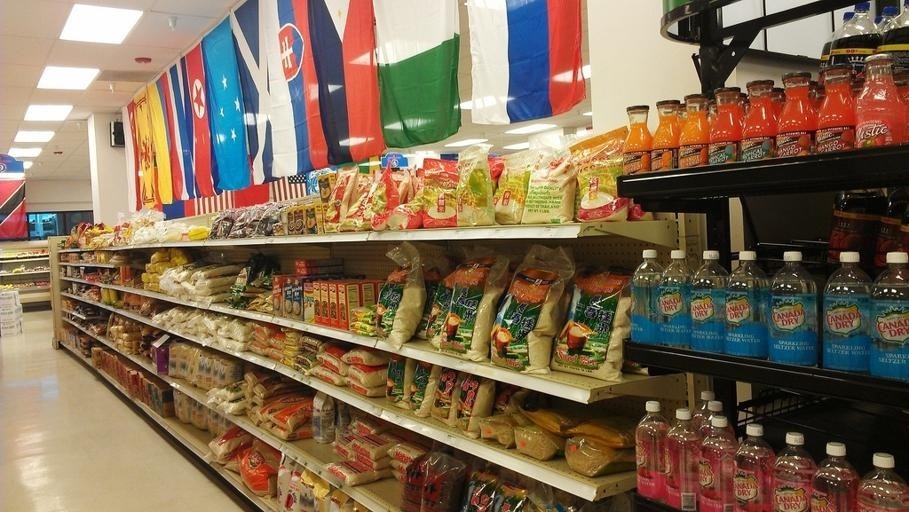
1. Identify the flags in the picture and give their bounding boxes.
[374,0,461,149]
[467,0,587,123]
[292,0,385,171]
[202,14,251,195]
[127,44,224,211]
[165,167,337,221]
[232,0,316,185]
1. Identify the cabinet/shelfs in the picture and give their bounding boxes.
[49,211,707,511]
[616,0,909,512]
[0,240,51,303]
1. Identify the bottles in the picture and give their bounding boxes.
[821,0,908,83]
[628,246,909,381]
[635,390,909,512]
[828,187,909,265]
[618,51,908,178]
